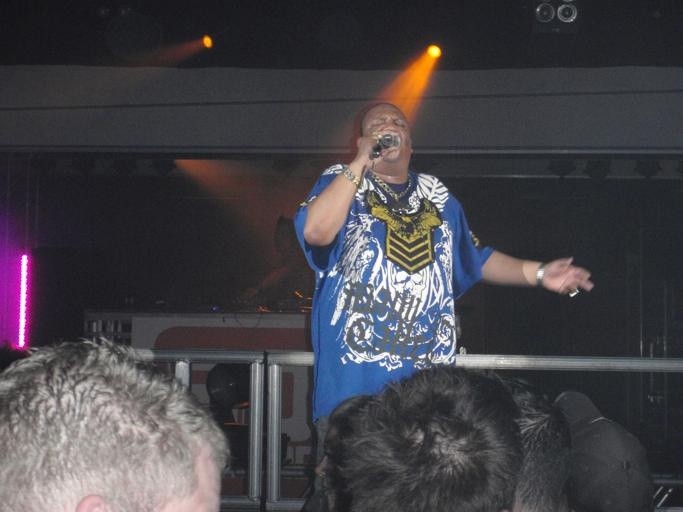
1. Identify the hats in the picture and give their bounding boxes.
[554,391,654,512]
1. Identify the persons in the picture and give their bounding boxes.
[1,337,230,512]
[294,103,596,512]
[302,367,654,512]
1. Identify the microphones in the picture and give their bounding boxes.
[381,133,399,147]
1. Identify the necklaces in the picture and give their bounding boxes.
[372,174,413,201]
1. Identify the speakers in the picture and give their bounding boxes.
[505,0,580,37]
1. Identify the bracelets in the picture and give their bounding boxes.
[341,168,360,189]
[535,262,547,287]
